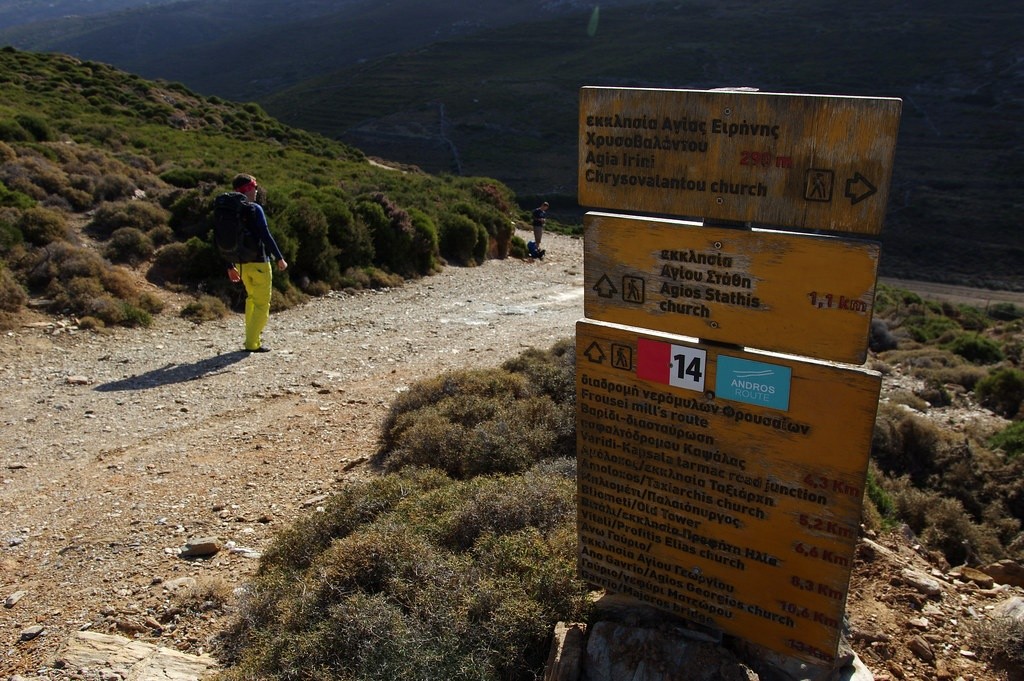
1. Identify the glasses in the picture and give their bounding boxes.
[253,185,258,190]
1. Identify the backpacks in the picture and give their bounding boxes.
[211,190,263,265]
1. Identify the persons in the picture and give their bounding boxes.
[213,173,287,352]
[532,202,549,251]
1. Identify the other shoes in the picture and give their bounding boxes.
[245,346,271,352]
